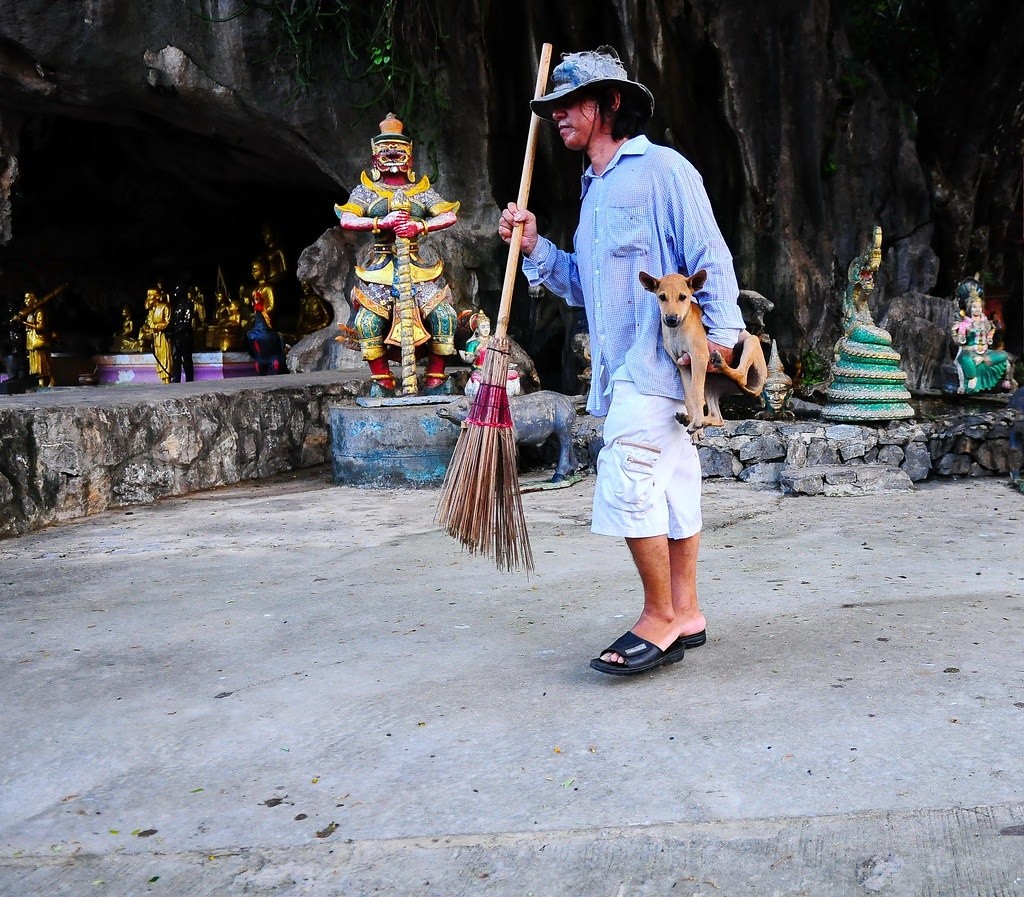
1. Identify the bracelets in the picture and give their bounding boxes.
[418,220,429,236]
[371,217,380,234]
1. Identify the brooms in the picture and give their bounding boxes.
[430,45,555,581]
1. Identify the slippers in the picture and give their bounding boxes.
[590,631,685,676]
[680,630,706,650]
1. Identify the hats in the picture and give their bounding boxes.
[529,45,655,123]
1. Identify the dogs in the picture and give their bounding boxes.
[637,270,768,444]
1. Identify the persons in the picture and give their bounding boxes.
[755,339,794,421]
[332,112,461,399]
[459,309,518,387]
[496,44,745,676]
[952,278,1015,393]
[13,292,56,386]
[115,253,331,385]
